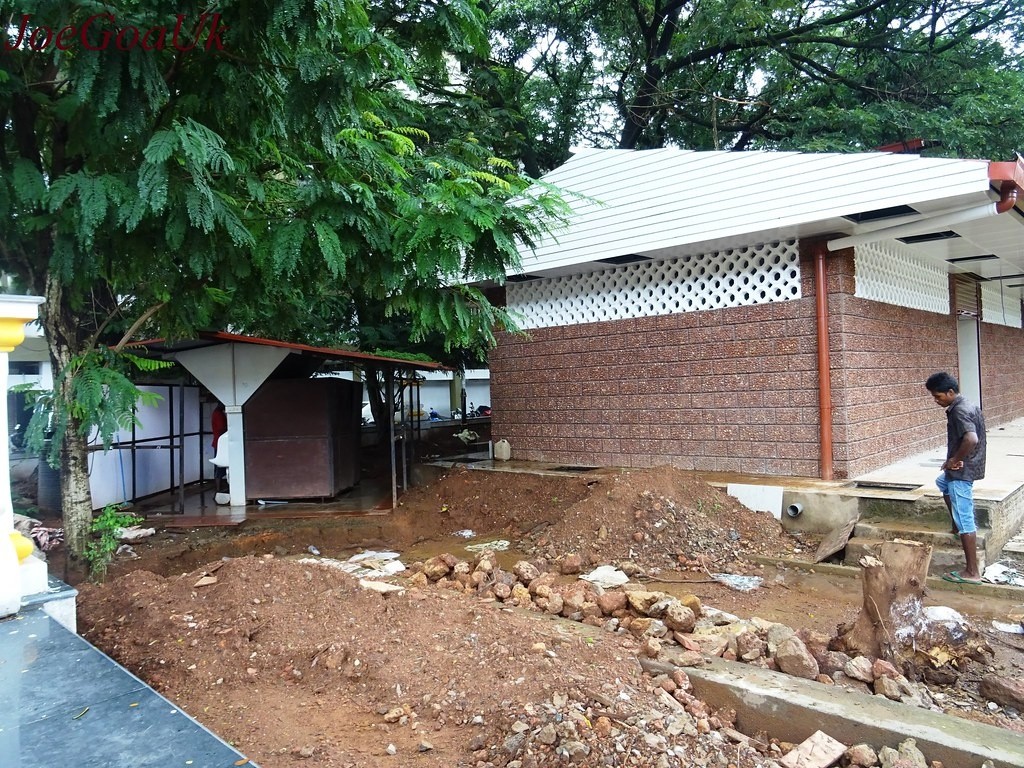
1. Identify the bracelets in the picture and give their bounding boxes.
[949,458,956,467]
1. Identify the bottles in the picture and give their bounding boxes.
[493,438,510,460]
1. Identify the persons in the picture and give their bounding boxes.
[925,372,986,585]
[212,401,227,494]
[9,424,27,454]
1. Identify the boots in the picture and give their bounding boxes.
[216,477,226,493]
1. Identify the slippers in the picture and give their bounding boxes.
[943,572,982,585]
[954,534,959,540]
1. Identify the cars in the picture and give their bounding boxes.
[361,399,492,426]
[9,422,42,488]
